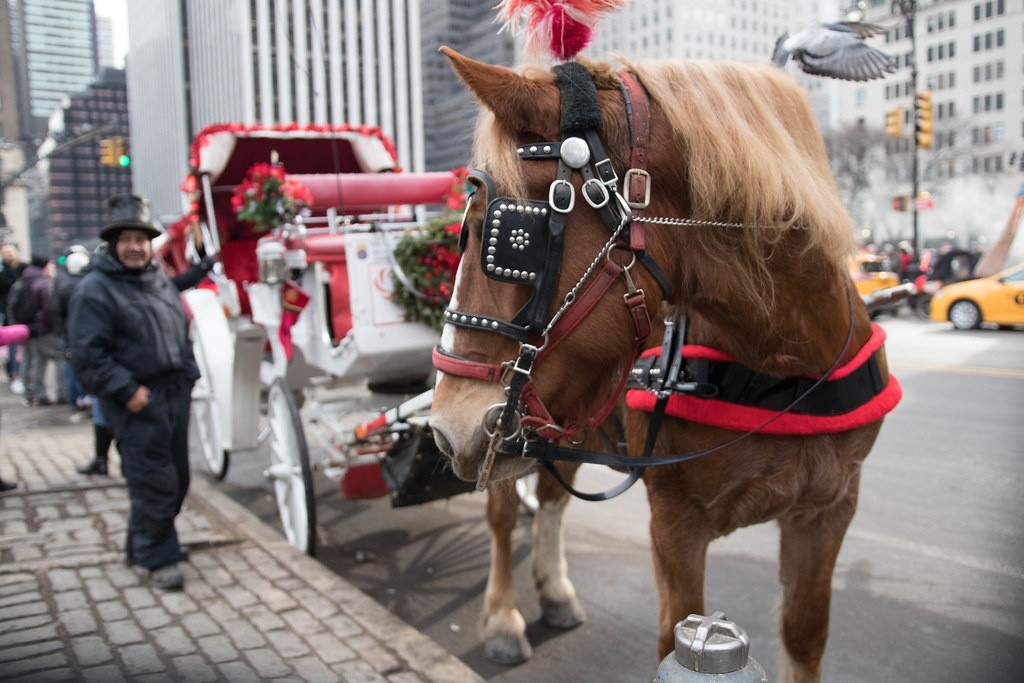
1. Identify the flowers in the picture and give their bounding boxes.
[388,166,481,326]
[231,162,289,234]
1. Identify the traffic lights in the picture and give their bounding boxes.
[888,91,933,147]
[100,138,131,168]
[57,248,66,272]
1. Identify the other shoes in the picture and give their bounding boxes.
[166,544,189,566]
[77,457,108,476]
[130,558,183,590]
[0,478,18,493]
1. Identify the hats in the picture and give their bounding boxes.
[96,194,158,242]
[33,254,48,266]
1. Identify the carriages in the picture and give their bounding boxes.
[151,41,891,683]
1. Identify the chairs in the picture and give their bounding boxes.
[199,187,293,355]
[294,170,485,344]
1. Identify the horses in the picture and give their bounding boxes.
[425,45,891,683]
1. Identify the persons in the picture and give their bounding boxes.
[842,231,985,316]
[0,237,227,491]
[65,192,201,594]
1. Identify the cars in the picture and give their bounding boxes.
[927,262,1024,330]
[844,254,899,316]
